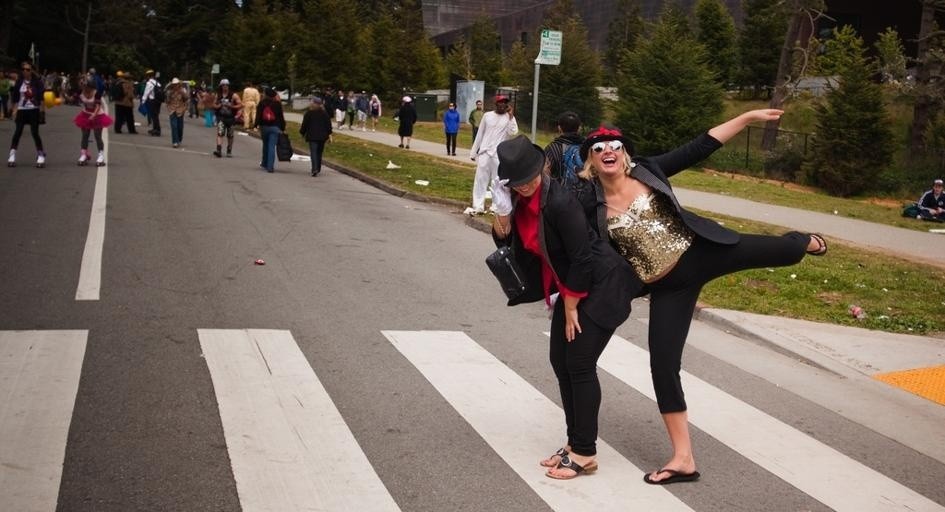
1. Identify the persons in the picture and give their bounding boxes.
[489,134,648,479]
[1,60,385,178]
[470,93,522,217]
[573,106,829,487]
[916,178,944,224]
[444,101,459,157]
[469,100,485,147]
[394,95,417,149]
[544,110,587,196]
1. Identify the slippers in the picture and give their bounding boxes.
[541,447,598,480]
[645,469,699,483]
[807,234,826,256]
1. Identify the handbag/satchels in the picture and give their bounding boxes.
[904,204,921,217]
[277,134,293,161]
[486,235,527,301]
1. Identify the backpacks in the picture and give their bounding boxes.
[150,80,164,103]
[562,144,598,209]
[109,81,127,101]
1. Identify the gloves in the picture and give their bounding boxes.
[489,176,513,217]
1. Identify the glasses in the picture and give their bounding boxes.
[592,140,623,153]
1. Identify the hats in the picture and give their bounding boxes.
[403,97,411,102]
[220,79,229,85]
[580,127,635,163]
[264,88,277,97]
[172,78,180,85]
[933,180,943,185]
[497,135,546,187]
[495,95,509,103]
[310,98,321,103]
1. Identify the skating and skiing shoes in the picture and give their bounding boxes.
[77,150,87,165]
[96,151,105,167]
[8,149,16,167]
[36,152,45,167]
